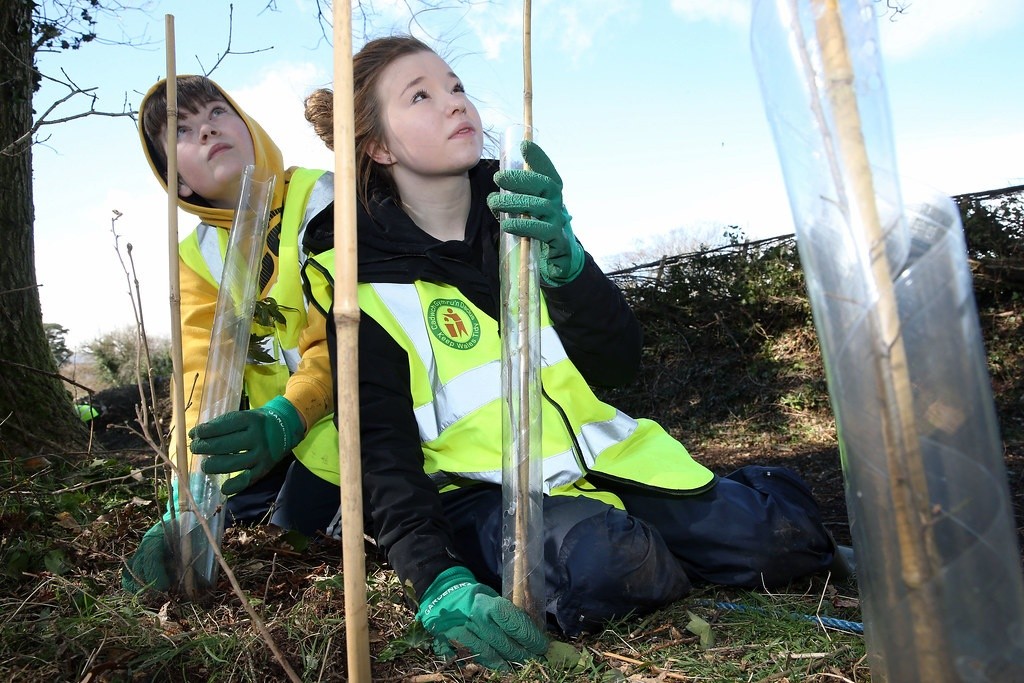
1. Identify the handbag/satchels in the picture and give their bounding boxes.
[723,466,836,595]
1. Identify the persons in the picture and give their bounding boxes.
[308,36,850,664]
[125,77,343,605]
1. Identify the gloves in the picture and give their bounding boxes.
[188,394,306,496]
[121,474,217,599]
[483,140,586,288]
[413,565,549,673]
